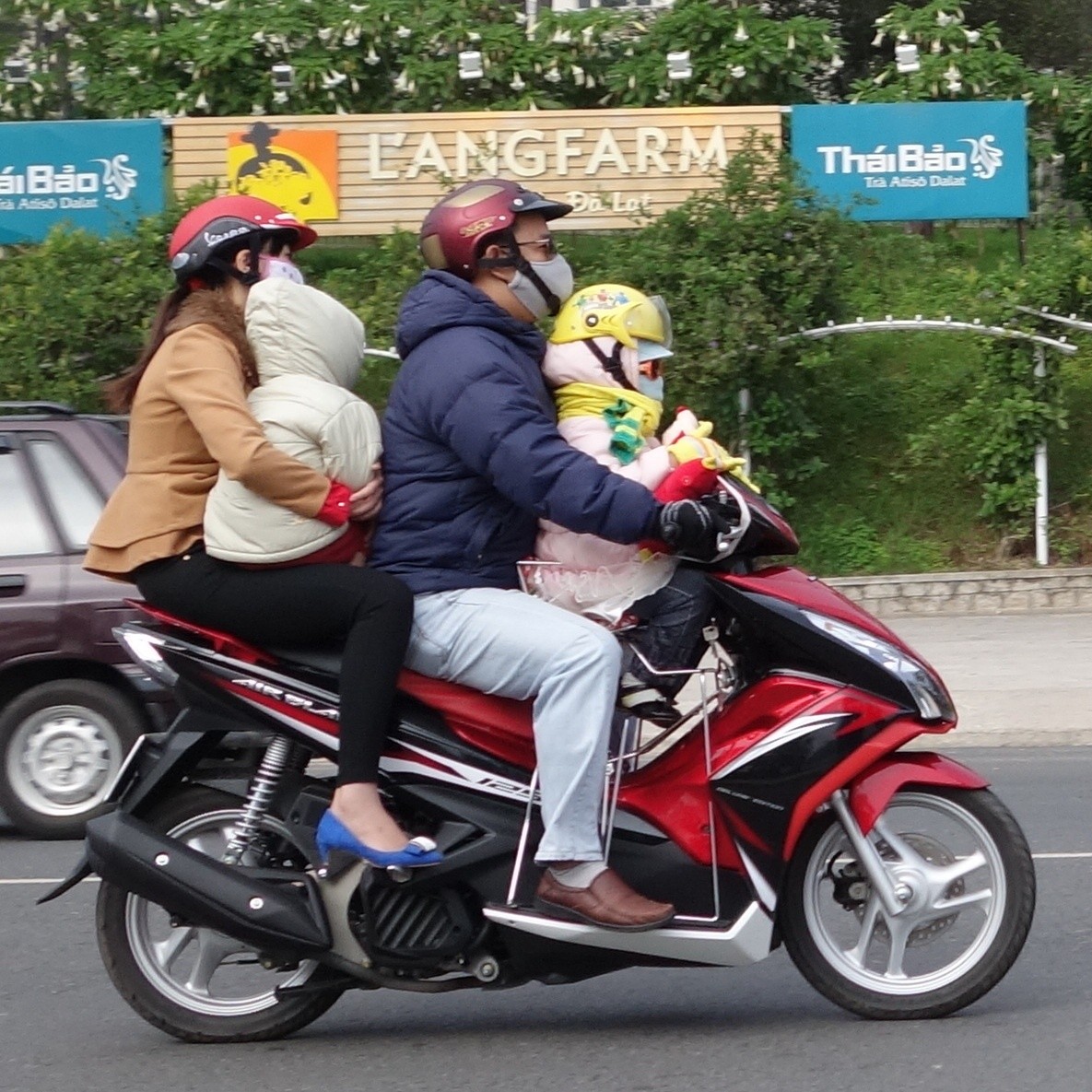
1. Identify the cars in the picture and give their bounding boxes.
[0,399,172,846]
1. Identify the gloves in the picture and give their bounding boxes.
[654,498,731,554]
[667,416,747,474]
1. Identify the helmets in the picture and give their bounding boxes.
[420,178,574,282]
[169,195,319,284]
[549,283,666,350]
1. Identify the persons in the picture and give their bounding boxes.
[527,284,730,729]
[203,276,385,565]
[83,199,443,868]
[369,179,731,932]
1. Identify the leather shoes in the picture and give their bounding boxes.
[616,677,682,731]
[533,868,678,934]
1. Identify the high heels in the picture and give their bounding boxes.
[315,808,447,869]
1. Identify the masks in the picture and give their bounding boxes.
[229,255,306,288]
[638,375,668,403]
[490,248,576,320]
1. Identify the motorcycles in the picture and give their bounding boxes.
[35,464,1040,1049]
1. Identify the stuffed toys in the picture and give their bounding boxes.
[663,407,760,493]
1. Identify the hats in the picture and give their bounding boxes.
[635,338,674,363]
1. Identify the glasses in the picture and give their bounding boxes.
[476,238,556,261]
